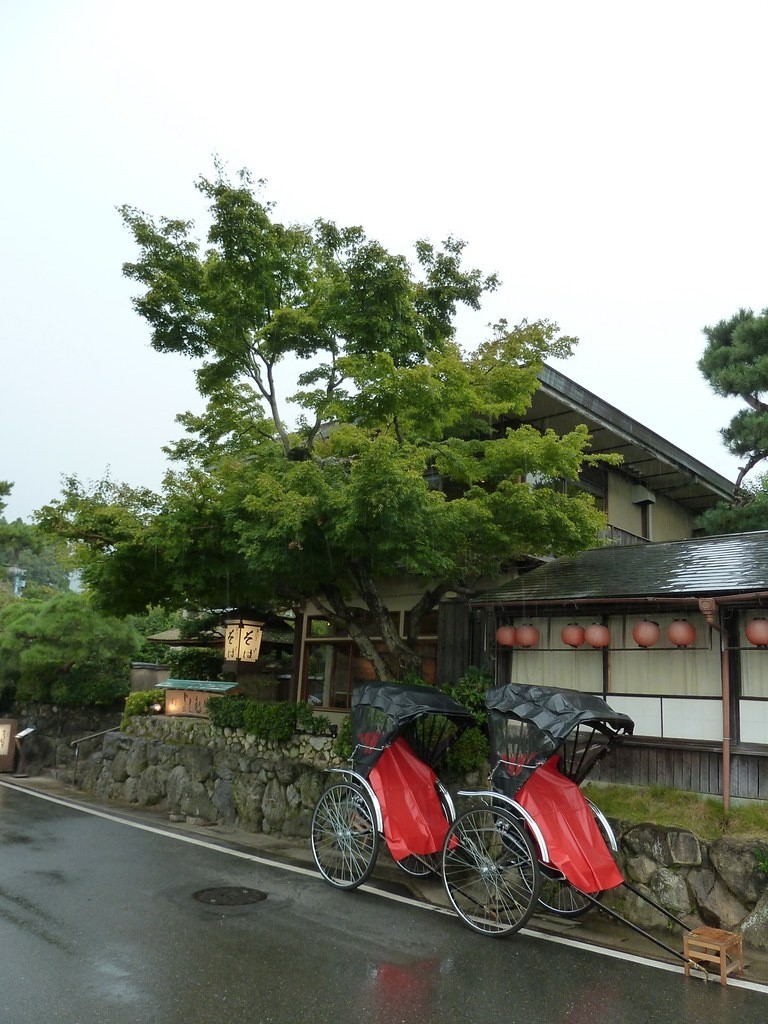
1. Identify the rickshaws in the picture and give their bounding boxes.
[438,682,745,981]
[308,677,625,929]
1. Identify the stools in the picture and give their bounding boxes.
[683,925,745,986]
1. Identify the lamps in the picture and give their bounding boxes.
[220,603,267,664]
[497,614,516,649]
[585,613,612,647]
[516,614,539,648]
[632,613,660,648]
[668,613,696,648]
[746,607,768,649]
[149,703,162,712]
[562,615,585,649]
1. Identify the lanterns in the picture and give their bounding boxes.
[745,617,768,647]
[667,618,696,647]
[515,622,540,647]
[561,623,586,648]
[585,622,610,648]
[632,619,660,648]
[496,623,517,647]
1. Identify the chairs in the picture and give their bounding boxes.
[500,752,584,852]
[356,730,434,823]
